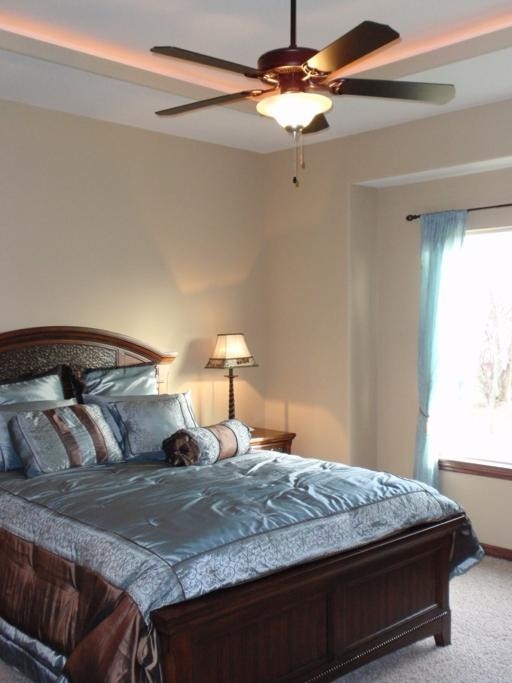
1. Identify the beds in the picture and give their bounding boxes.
[0,325,484,683]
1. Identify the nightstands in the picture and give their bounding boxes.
[243,427,296,454]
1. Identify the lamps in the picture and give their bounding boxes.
[205,333,258,419]
[255,92,332,184]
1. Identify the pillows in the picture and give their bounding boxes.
[0,363,252,477]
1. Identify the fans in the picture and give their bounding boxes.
[150,0,455,133]
